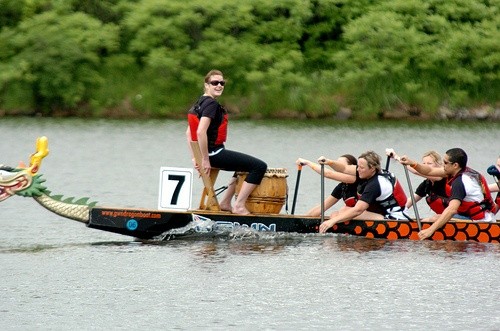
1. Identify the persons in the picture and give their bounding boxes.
[296,147,500,242]
[186,69,268,214]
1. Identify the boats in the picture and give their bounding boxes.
[0,135,500,244]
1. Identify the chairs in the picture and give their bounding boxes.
[190,140,236,213]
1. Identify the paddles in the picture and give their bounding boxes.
[386,153,393,170]
[291,162,304,215]
[321,159,325,224]
[492,168,500,189]
[402,158,422,231]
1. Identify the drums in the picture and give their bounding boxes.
[237,168,289,215]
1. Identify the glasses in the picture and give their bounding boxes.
[443,160,455,164]
[206,80,225,86]
